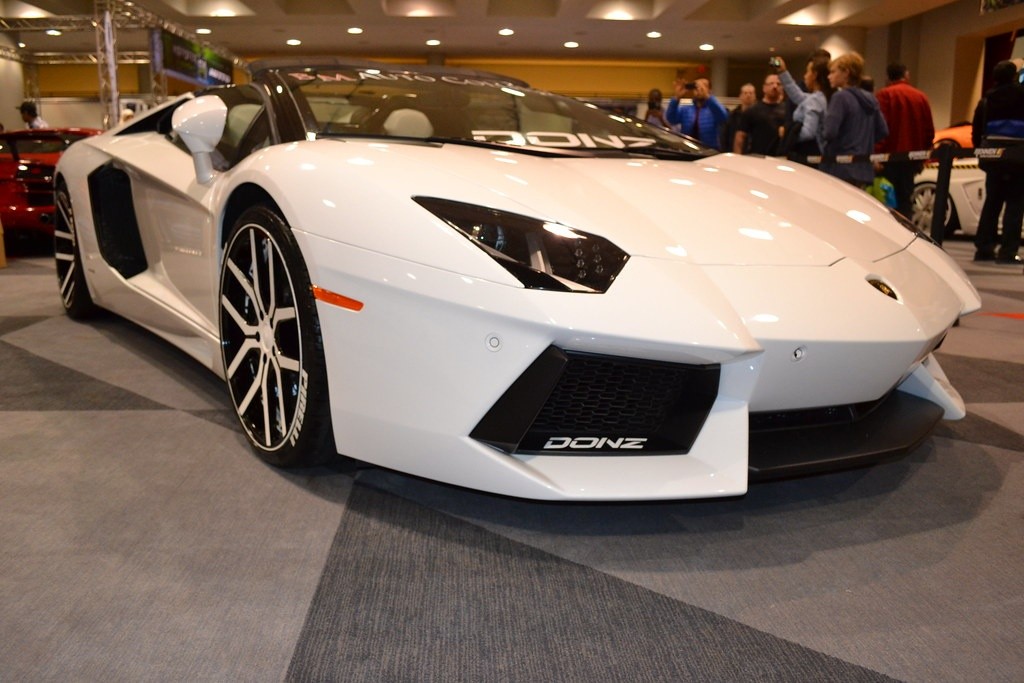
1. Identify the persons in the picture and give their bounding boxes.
[645,90,676,131]
[719,48,890,193]
[874,62,934,221]
[971,59,1024,265]
[666,78,729,151]
[15,101,49,131]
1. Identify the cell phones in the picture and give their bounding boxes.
[685,83,696,90]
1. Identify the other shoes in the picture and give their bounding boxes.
[973,249,1023,264]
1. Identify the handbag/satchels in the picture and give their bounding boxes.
[862,167,898,211]
[978,134,1024,174]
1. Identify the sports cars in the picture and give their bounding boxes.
[52,62,983,502]
[0,128,108,253]
[910,121,1024,239]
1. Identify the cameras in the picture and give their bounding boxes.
[770,57,780,67]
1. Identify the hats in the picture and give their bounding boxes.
[15,101,36,110]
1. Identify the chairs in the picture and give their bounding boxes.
[228,104,269,151]
[383,108,435,139]
